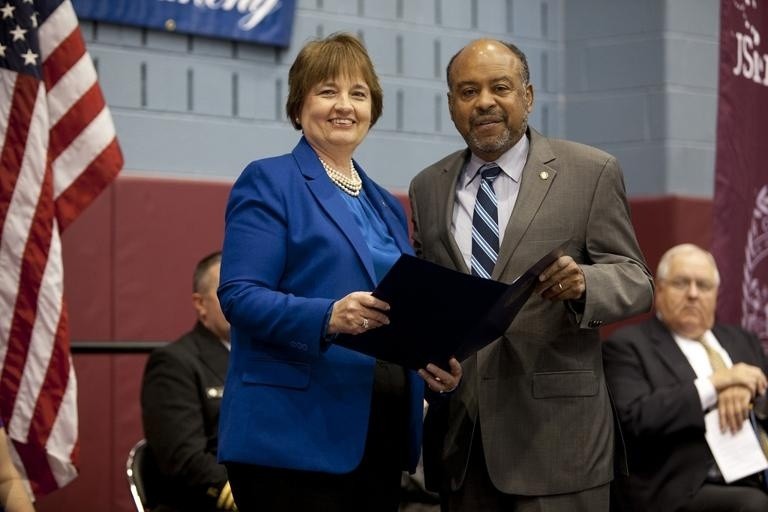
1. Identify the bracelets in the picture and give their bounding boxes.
[0,476,22,483]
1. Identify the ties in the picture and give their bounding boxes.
[469,167,504,279]
[696,335,767,460]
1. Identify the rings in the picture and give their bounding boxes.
[362,317,370,328]
[557,282,564,292]
[439,387,445,394]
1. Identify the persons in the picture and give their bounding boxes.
[0,425,37,511]
[138,250,245,510]
[406,34,656,511]
[600,235,768,511]
[222,27,467,511]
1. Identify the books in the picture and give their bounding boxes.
[703,402,768,486]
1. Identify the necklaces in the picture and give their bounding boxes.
[317,154,363,198]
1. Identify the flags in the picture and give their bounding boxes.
[0,2,125,494]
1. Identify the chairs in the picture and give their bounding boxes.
[126,437,150,512]
